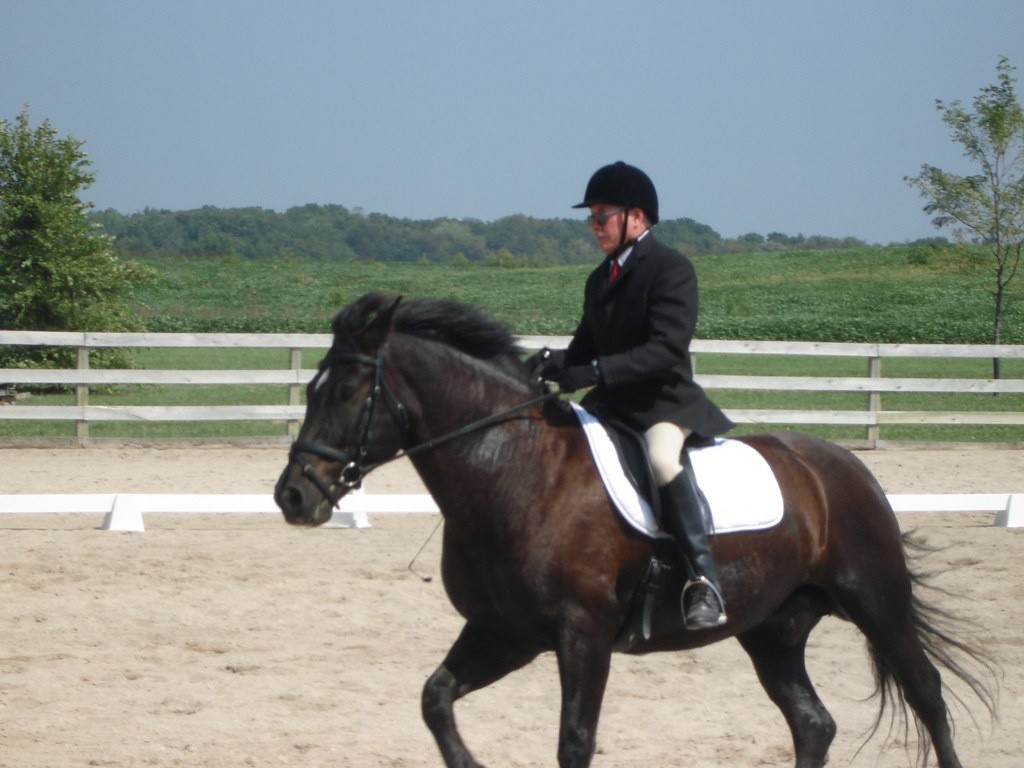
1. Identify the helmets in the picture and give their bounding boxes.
[571,161,658,213]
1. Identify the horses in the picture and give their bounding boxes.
[272,289,1007,768]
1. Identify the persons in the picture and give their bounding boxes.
[525,162,737,629]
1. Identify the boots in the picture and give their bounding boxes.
[656,468,723,630]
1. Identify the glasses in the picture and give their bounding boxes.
[587,207,625,226]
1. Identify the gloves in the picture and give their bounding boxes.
[525,348,600,393]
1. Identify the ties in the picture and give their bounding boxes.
[608,261,620,286]
